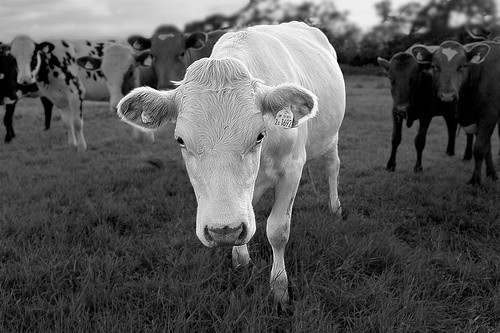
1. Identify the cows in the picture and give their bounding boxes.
[377,42,470,172]
[5,34,130,152]
[412,39,500,188]
[120,50,165,144]
[116,20,347,312]
[128,25,242,91]
[0,39,55,140]
